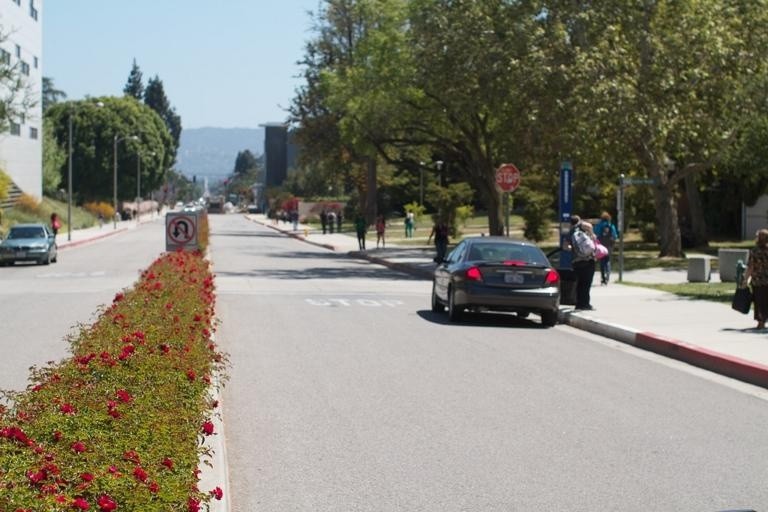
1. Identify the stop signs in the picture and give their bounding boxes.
[495,161,521,192]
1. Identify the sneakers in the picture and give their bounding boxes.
[575,304,596,312]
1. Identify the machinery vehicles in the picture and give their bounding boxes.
[206,196,224,214]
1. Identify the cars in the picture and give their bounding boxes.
[184,204,195,212]
[0,224,57,266]
[432,236,579,326]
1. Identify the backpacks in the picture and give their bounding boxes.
[571,231,595,258]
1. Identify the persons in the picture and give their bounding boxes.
[97,212,104,230]
[561,213,598,311]
[374,210,387,251]
[50,210,60,237]
[427,215,450,266]
[273,206,344,235]
[353,211,369,251]
[404,209,417,238]
[739,229,768,330]
[592,211,618,285]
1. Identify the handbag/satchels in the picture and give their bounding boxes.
[594,238,609,261]
[731,286,753,315]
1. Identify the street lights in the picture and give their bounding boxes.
[435,159,444,214]
[68,102,104,241]
[114,136,138,229]
[137,152,156,222]
[419,160,426,206]
